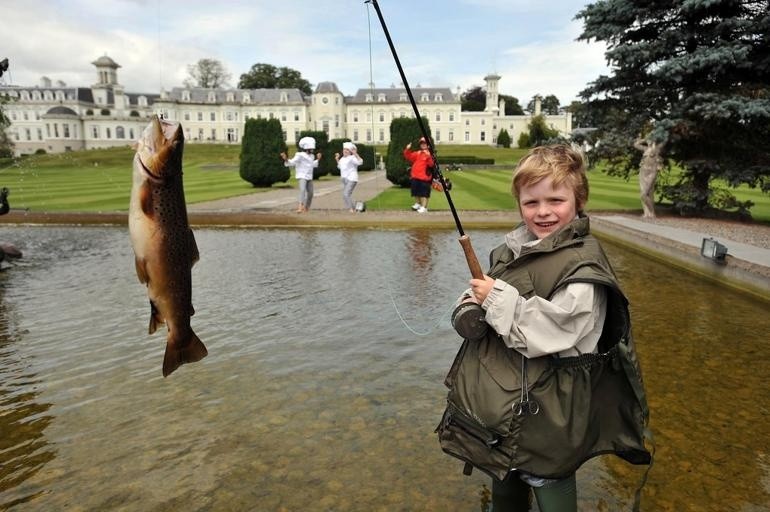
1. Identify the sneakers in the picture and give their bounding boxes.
[411,202,422,210]
[303,207,309,214]
[416,207,427,214]
[350,207,357,216]
[296,202,303,213]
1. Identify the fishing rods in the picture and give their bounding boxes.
[365,0,495,338]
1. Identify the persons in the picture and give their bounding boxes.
[280,136,322,212]
[451,144,653,511]
[336,141,363,212]
[404,136,435,213]
[632,131,669,218]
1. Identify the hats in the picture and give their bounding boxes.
[419,137,426,143]
[342,141,357,154]
[299,137,316,150]
